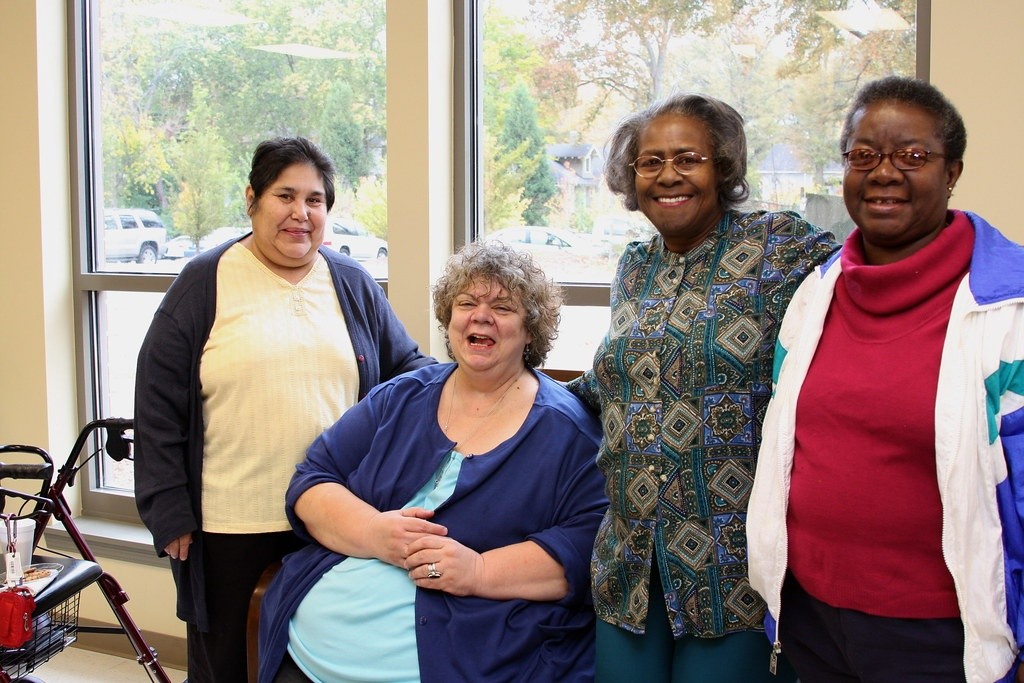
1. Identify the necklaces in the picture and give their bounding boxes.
[434,366,512,489]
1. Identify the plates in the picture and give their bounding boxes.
[0,563,64,586]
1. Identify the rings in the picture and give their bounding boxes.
[427,563,441,578]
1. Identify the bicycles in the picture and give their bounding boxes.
[1,415,174,683]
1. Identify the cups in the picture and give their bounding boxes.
[0,519,36,570]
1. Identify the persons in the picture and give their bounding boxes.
[557,90,842,683]
[134,135,442,683]
[256,239,609,683]
[743,73,1024,683]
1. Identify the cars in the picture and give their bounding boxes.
[161,235,195,262]
[198,226,254,254]
[482,228,611,282]
[321,218,388,260]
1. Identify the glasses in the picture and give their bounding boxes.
[629,152,716,178]
[842,148,949,171]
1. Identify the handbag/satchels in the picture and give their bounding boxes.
[0,584,36,649]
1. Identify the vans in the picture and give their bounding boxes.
[105,208,167,266]
